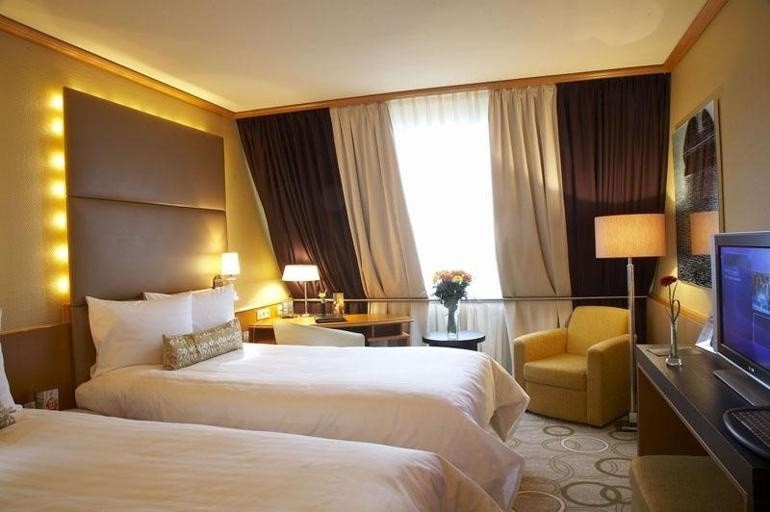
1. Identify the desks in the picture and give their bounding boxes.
[247,313,415,347]
[635,344,769,512]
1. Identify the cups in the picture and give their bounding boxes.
[333,292,345,316]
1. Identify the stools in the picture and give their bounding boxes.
[629,455,744,511]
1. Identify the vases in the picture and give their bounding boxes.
[445,305,458,339]
[665,323,682,366]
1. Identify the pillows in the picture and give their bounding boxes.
[85,291,193,378]
[161,318,243,370]
[143,283,235,336]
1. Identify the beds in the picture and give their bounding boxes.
[1,408,503,512]
[74,342,531,511]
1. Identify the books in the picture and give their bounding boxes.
[315,315,346,322]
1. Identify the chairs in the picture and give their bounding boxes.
[272,319,365,347]
[512,305,638,428]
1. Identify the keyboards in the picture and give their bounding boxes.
[722,407,770,459]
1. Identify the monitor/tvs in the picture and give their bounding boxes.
[710,231,770,408]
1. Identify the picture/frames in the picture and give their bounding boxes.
[670,93,724,291]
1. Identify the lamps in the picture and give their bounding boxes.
[594,213,668,433]
[222,252,240,281]
[282,264,320,318]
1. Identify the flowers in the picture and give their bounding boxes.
[660,275,680,358]
[430,269,472,333]
